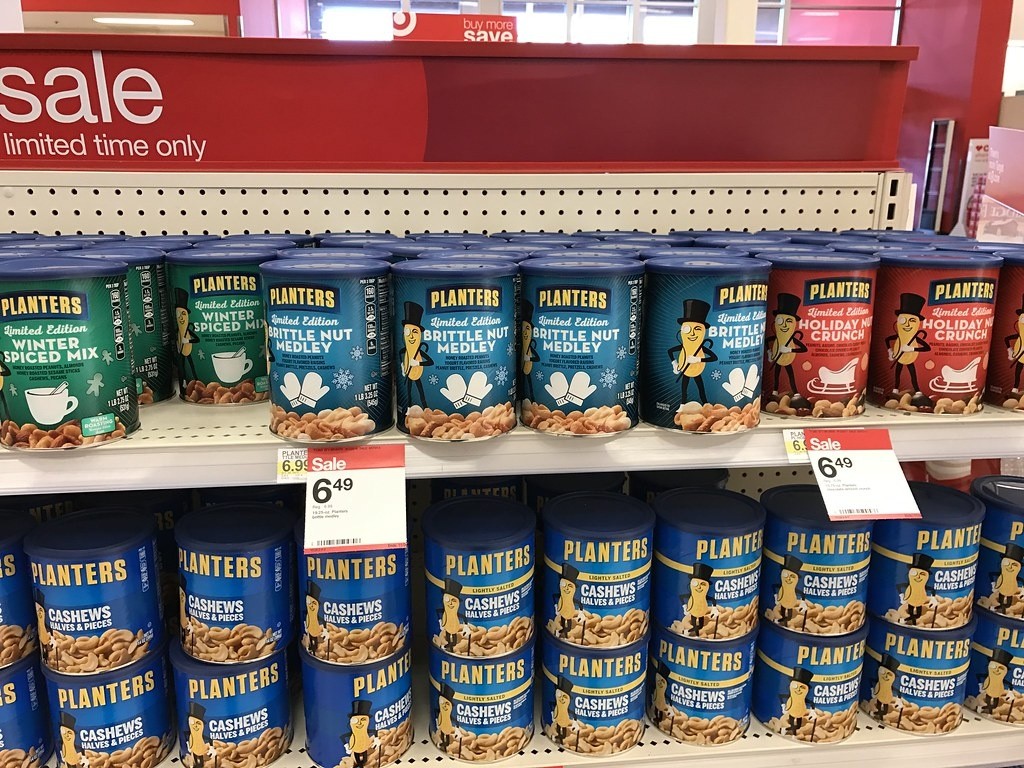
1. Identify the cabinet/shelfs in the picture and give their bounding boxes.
[0,164,1024,768]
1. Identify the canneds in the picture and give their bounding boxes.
[0,231,1024,768]
